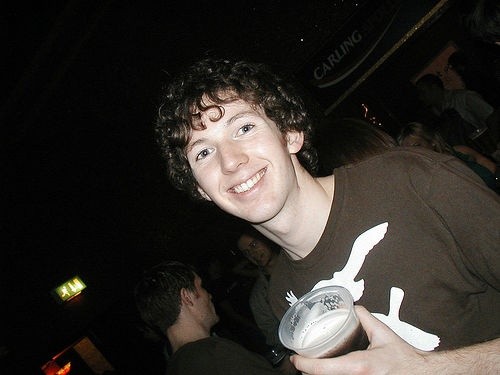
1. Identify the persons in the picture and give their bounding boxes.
[399,121,499,195]
[412,74,495,154]
[235,232,282,347]
[126,259,277,375]
[155,58,500,375]
[314,115,397,172]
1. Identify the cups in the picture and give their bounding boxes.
[279,285,371,359]
